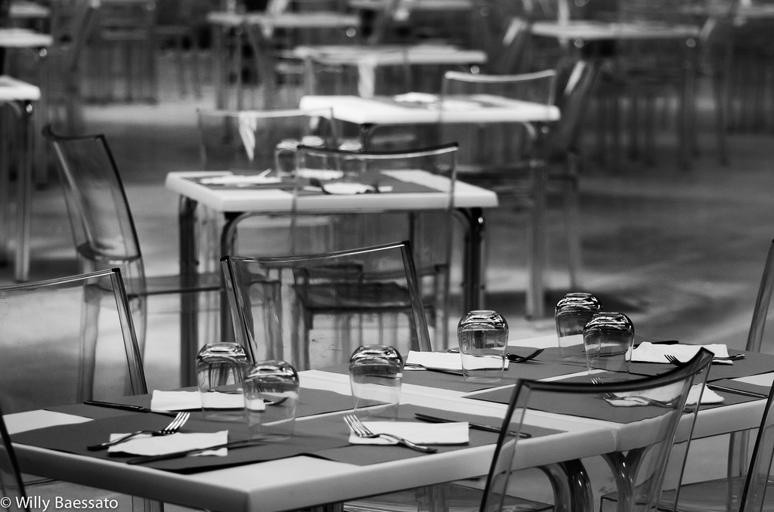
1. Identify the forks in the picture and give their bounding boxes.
[447,347,546,363]
[663,351,746,367]
[341,414,439,457]
[587,375,686,410]
[84,410,193,453]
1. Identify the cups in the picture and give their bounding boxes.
[348,342,402,423]
[456,307,507,385]
[273,145,367,187]
[586,310,637,385]
[196,340,250,424]
[553,289,603,364]
[240,359,301,446]
